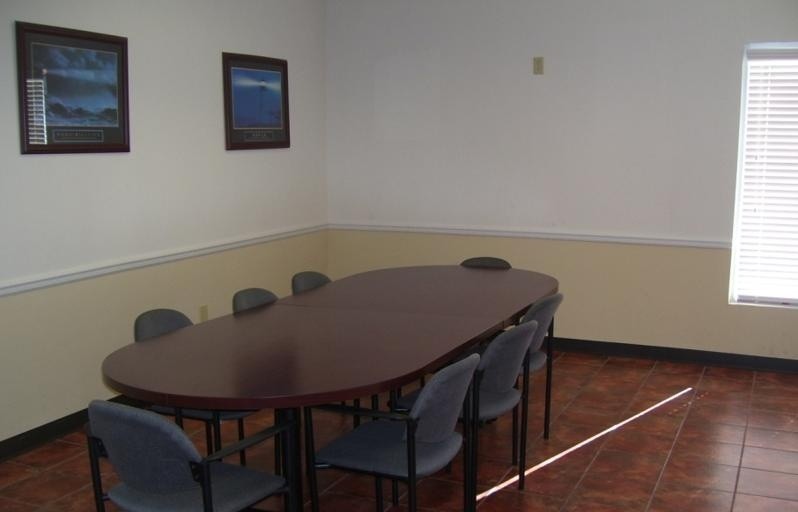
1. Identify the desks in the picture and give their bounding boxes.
[102,265,558,511]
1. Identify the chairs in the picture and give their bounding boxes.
[86,398,299,511]
[386,321,539,492]
[493,292,565,466]
[133,307,249,470]
[232,288,320,482]
[314,353,482,511]
[460,256,513,268]
[292,270,404,423]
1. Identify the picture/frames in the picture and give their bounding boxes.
[223,49,292,150]
[16,20,132,155]
